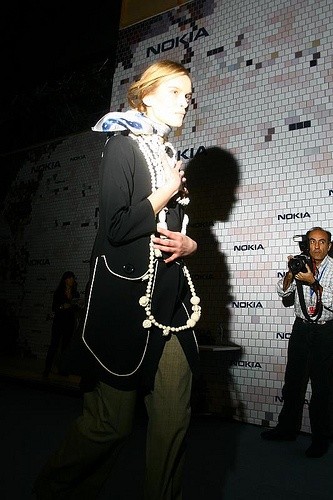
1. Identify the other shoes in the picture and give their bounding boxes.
[305,444,328,457]
[260,429,294,442]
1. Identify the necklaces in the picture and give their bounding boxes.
[127,133,201,335]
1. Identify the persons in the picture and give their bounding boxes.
[42,270,84,377]
[260,227,333,458]
[31,59,202,500]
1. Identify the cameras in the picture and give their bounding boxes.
[288,235,313,274]
